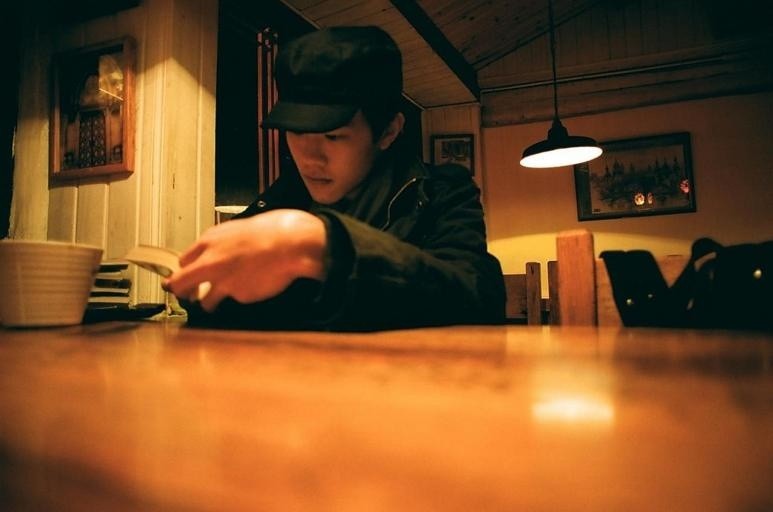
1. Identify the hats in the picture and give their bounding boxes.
[259,25,403,135]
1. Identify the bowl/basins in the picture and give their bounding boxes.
[0,241,103,329]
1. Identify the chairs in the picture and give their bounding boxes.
[505,227,710,326]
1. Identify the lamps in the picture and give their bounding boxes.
[517,1,604,170]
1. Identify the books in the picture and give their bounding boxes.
[125,242,213,301]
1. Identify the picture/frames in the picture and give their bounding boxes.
[49,34,136,181]
[428,131,475,179]
[572,131,697,223]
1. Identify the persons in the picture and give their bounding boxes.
[161,25,506,332]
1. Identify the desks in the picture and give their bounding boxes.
[0,319,772,512]
